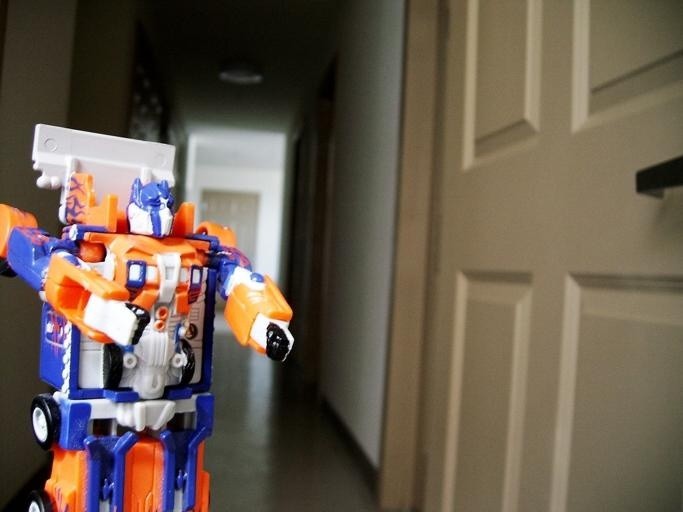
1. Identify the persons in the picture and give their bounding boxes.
[1,122,296,512]
